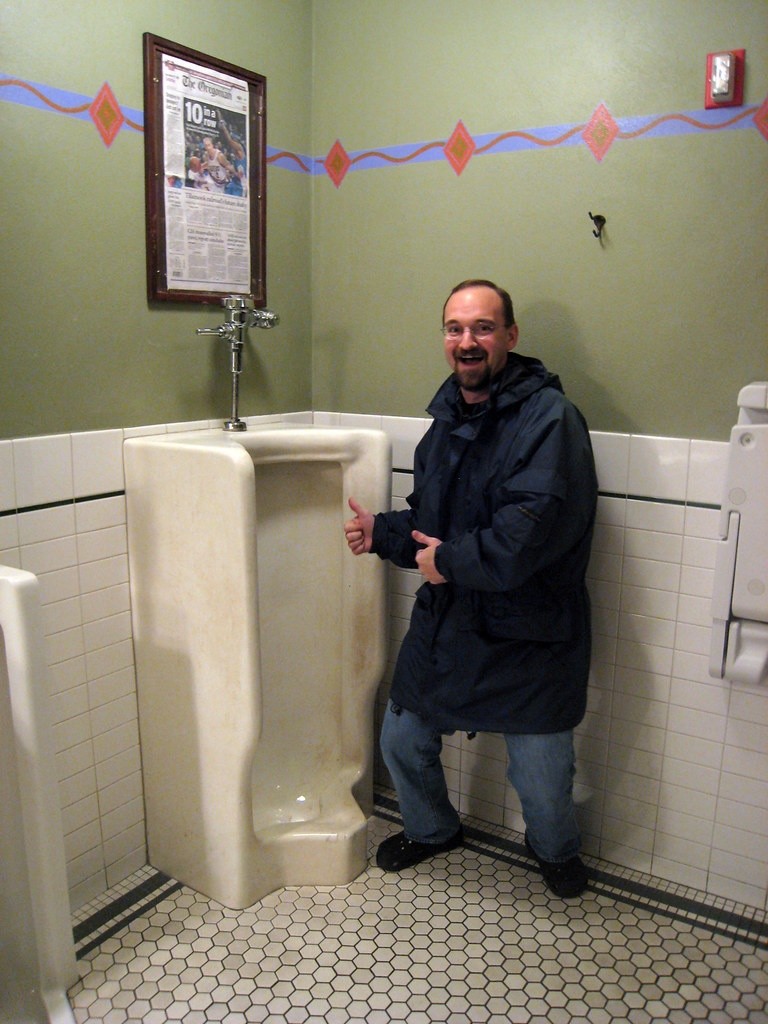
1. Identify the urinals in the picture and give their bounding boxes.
[1,566,81,1023]
[124,426,391,912]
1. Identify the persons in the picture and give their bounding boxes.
[189,110,246,196]
[344,279,598,897]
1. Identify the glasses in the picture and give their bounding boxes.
[440,323,505,340]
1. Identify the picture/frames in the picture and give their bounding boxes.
[141,31,269,311]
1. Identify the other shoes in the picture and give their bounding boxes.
[525,829,588,897]
[376,823,463,872]
